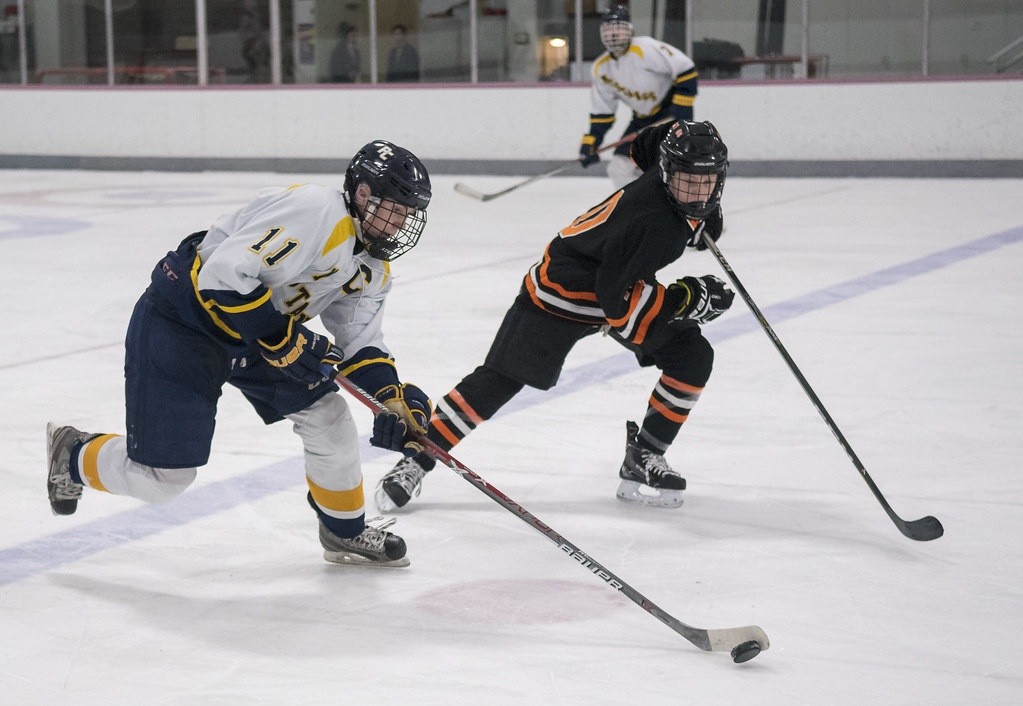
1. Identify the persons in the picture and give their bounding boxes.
[240,0,291,83]
[46,139,432,567]
[331,23,361,83]
[376,119,736,514]
[387,24,420,82]
[579,5,700,188]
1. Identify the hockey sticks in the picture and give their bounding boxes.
[454,115,675,203]
[701,228,945,542]
[319,362,774,656]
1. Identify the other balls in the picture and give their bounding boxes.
[730,640,761,664]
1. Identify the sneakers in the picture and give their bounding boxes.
[616,421,686,509]
[307,492,411,567]
[46,420,106,515]
[374,453,436,514]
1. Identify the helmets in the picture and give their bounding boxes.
[342,140,432,260]
[657,119,730,221]
[602,5,628,22]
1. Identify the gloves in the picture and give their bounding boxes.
[369,383,433,456]
[667,275,735,325]
[579,134,603,167]
[256,314,344,391]
[686,204,723,251]
